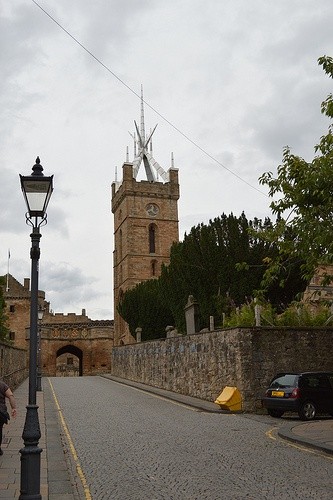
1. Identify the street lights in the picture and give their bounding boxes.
[18,156,54,500]
[36,304,46,391]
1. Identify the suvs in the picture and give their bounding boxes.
[261,370,333,422]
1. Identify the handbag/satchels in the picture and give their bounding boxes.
[3,412,10,424]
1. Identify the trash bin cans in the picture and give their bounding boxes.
[214,385,243,410]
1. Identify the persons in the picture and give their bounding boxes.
[0,381,17,455]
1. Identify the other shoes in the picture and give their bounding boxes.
[0,451,3,455]
